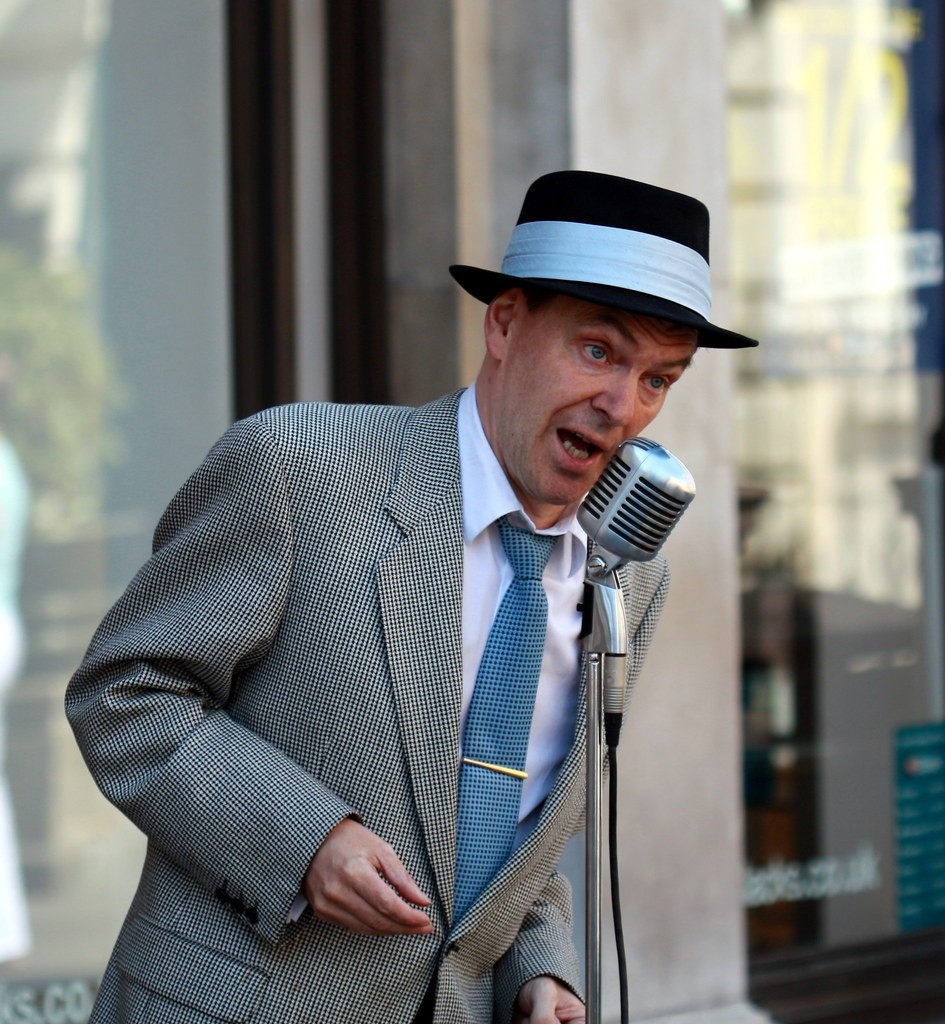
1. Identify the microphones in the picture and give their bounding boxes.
[577,436,697,747]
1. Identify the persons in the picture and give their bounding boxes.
[64,169,758,1024]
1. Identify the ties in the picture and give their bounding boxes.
[453,514,559,930]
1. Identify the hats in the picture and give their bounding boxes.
[449,170,759,349]
